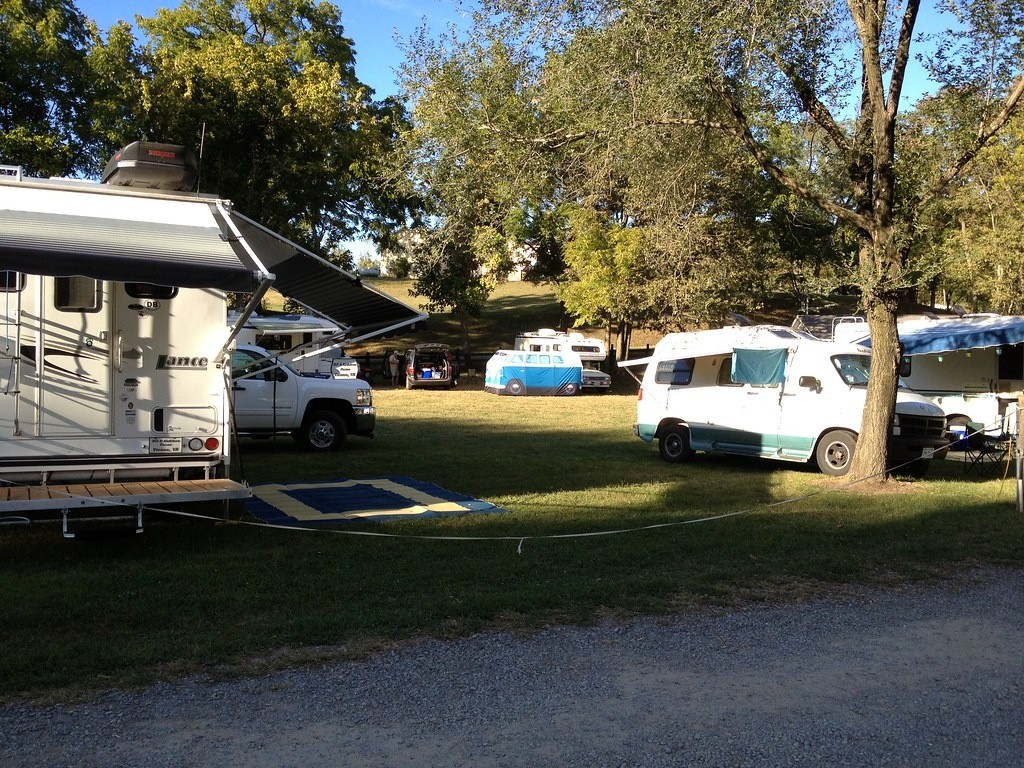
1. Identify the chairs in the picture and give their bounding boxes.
[962,422,1015,477]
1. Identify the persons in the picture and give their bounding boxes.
[389,350,401,389]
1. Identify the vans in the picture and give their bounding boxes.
[617,313,1024,476]
[514,328,611,392]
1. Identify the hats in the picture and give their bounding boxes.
[394,350,400,355]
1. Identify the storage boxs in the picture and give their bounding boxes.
[416,367,442,379]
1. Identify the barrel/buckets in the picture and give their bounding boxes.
[422,368,432,378]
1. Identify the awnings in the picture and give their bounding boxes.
[232,207,429,377]
[1,184,276,366]
[851,317,1024,355]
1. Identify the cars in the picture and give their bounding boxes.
[227,345,377,453]
[383,342,452,390]
